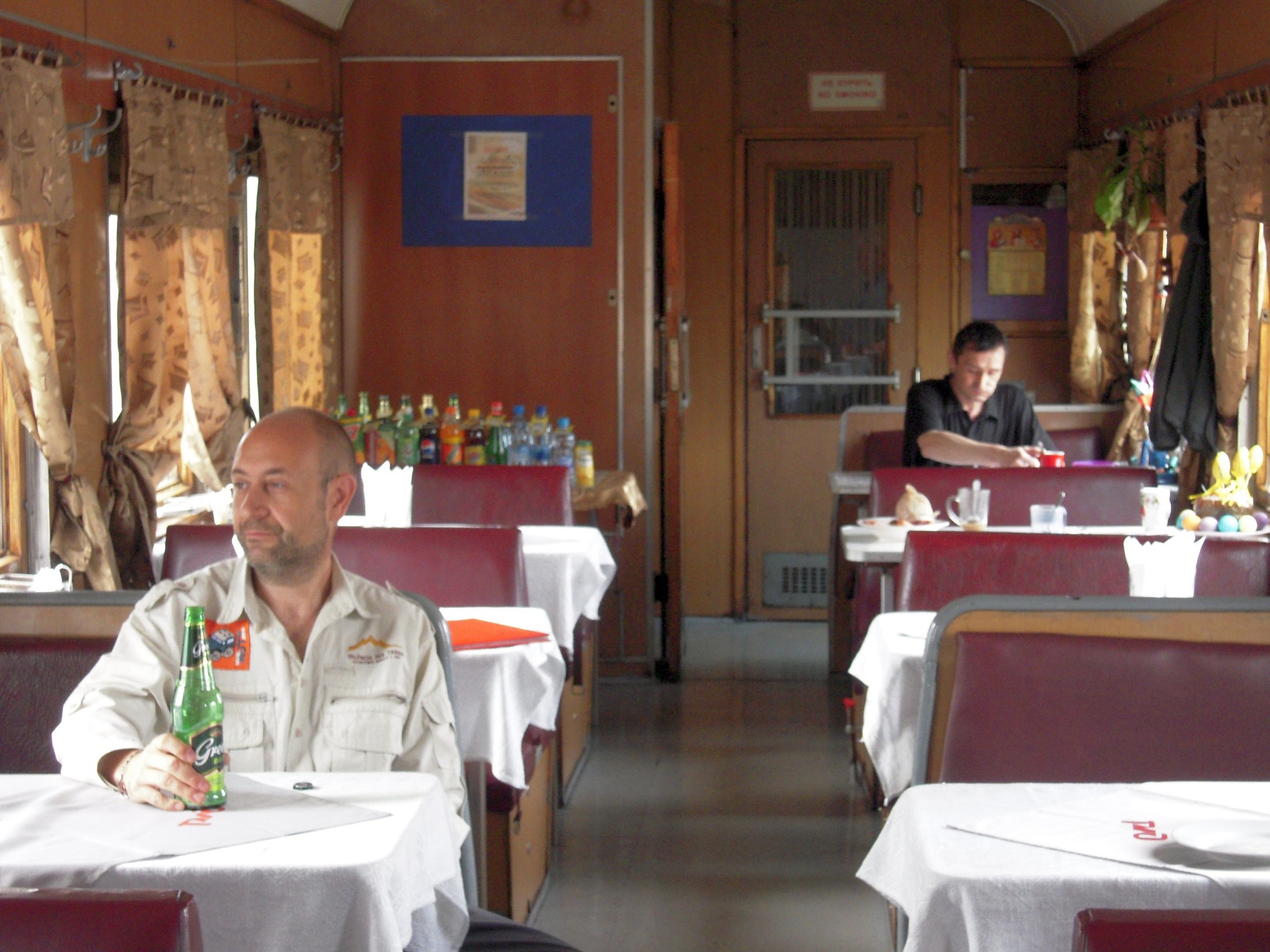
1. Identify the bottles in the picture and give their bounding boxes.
[551,418,577,485]
[485,401,509,465]
[529,405,552,466]
[575,439,595,488]
[171,607,227,809]
[438,393,463,466]
[395,394,417,464]
[418,394,438,463]
[357,390,373,465]
[463,407,485,466]
[509,405,529,467]
[373,393,395,468]
[334,393,357,456]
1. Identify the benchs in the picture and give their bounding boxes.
[0,402,1270,952]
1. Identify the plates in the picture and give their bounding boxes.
[1172,822,1270,865]
[1073,460,1119,466]
[855,516,950,533]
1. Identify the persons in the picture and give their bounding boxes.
[50,405,584,952]
[901,320,1056,467]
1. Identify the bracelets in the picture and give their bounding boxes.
[118,749,144,797]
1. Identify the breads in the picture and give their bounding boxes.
[894,483,934,524]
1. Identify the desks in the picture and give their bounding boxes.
[439,605,566,908]
[850,610,939,810]
[0,769,472,952]
[569,471,646,535]
[854,781,1270,952]
[339,514,614,657]
[840,523,1179,612]
[828,469,876,523]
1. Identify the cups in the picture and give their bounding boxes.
[945,486,991,532]
[1038,451,1066,467]
[1029,503,1069,533]
[1140,487,1172,526]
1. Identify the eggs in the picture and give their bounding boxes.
[1177,509,1268,533]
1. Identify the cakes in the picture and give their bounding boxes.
[1191,446,1263,518]
